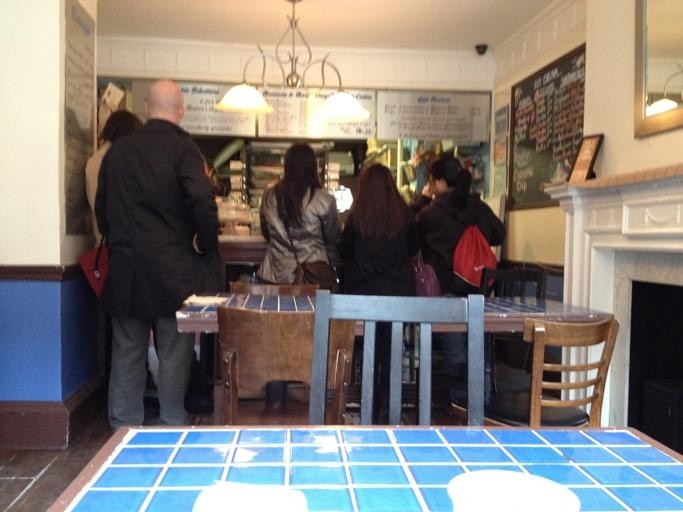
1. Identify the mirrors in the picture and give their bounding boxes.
[631,0,683,139]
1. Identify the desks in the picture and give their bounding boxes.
[199,233,419,386]
[48,425,683,512]
[176,295,364,403]
[432,296,613,397]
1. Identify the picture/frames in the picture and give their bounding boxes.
[566,133,605,183]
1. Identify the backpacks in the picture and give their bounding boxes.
[431,201,497,298]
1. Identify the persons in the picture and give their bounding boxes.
[85,109,144,248]
[408,153,499,414]
[337,164,421,423]
[256,143,342,293]
[94,79,215,428]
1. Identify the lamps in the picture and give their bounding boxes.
[210,0,372,129]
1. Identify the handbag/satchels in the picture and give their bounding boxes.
[78,230,108,295]
[283,219,334,293]
[413,248,441,296]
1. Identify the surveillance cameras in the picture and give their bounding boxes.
[475,44,488,54]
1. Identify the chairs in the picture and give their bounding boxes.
[310,290,485,430]
[231,282,320,296]
[216,311,357,426]
[450,316,620,429]
[456,266,547,394]
